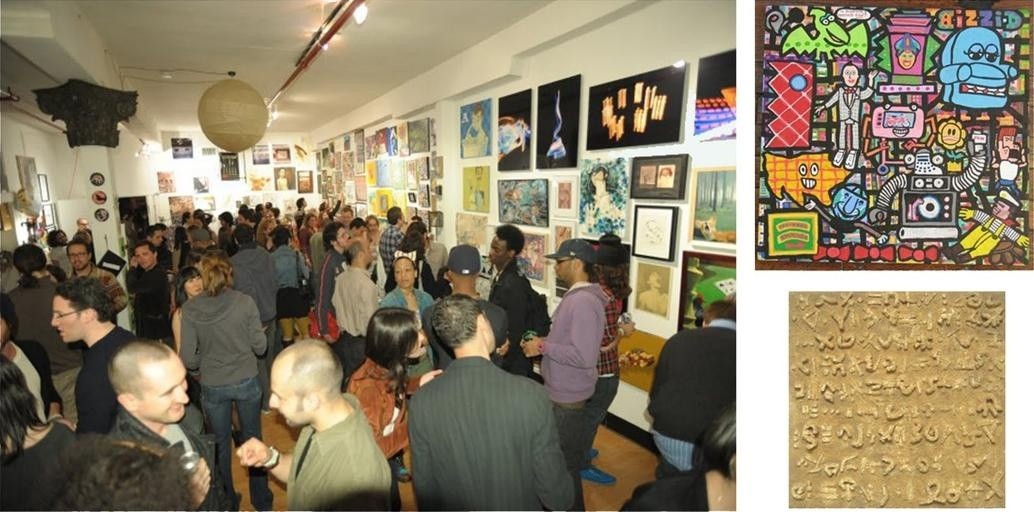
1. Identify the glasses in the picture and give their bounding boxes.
[53,308,85,321]
[556,258,572,264]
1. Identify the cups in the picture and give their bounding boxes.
[522,330,538,348]
[180,451,199,476]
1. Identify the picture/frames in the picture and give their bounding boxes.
[90,172,109,222]
[628,153,737,333]
[17,155,57,249]
[553,175,578,219]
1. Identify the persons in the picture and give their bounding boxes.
[1,199,735,511]
[277,168,289,190]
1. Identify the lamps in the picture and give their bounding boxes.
[198,71,269,155]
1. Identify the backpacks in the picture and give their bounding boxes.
[524,278,552,338]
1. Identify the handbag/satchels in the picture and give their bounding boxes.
[295,250,316,302]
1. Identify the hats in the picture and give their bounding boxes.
[543,238,598,265]
[596,234,627,265]
[448,244,481,276]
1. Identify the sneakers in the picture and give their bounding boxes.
[591,448,599,458]
[397,459,412,482]
[578,464,616,485]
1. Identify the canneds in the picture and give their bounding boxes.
[617,312,631,325]
[523,330,538,343]
[178,450,200,476]
